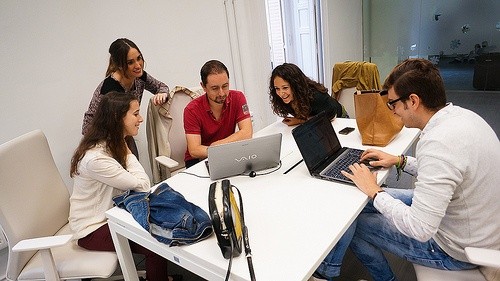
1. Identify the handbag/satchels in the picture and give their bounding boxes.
[208,179,242,259]
[354,89,404,147]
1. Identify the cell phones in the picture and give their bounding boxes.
[339,127,355,135]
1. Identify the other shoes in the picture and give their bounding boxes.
[167,274,183,281]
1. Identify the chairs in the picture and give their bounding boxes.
[145,86,207,185]
[0,129,146,281]
[412,247,500,281]
[331,62,383,119]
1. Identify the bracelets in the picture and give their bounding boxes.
[396,154,407,182]
[373,190,386,202]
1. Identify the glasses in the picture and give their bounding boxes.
[386,97,402,109]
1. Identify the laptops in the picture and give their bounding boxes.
[205,134,282,180]
[291,111,383,185]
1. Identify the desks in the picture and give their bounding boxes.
[105,112,422,281]
[429,55,475,68]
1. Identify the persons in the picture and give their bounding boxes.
[67,92,195,281]
[308,58,500,281]
[81,38,170,161]
[183,60,253,169]
[269,63,350,125]
